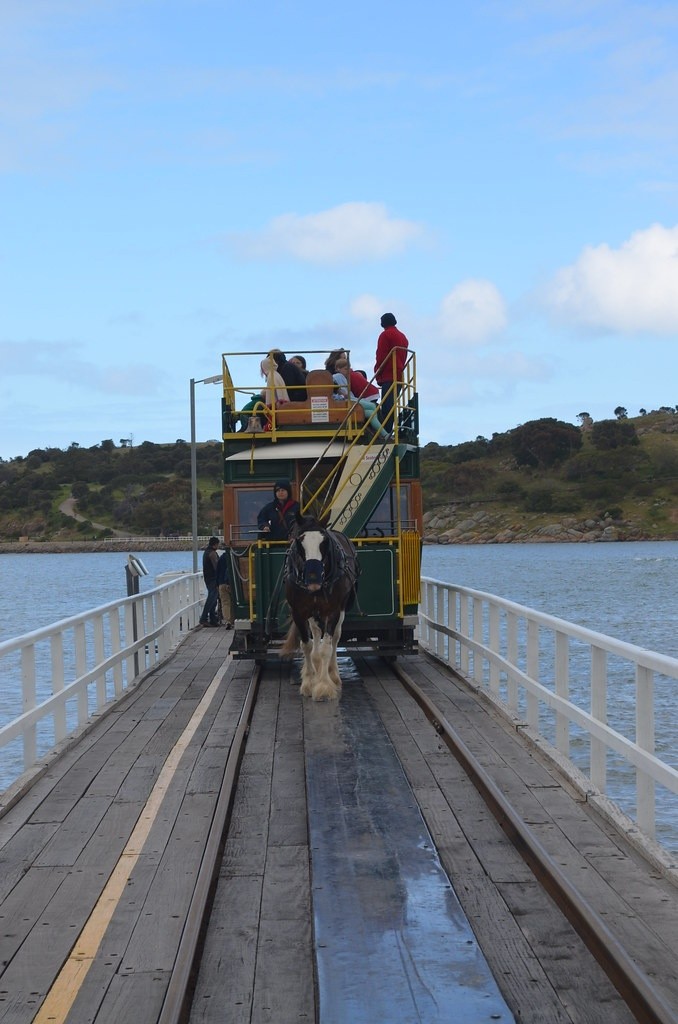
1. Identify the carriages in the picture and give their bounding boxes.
[223,346,425,704]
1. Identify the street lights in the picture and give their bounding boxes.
[190,374,224,573]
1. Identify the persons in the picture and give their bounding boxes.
[199,537,234,631]
[256,479,299,541]
[236,313,408,442]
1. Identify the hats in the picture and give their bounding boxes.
[266,349,284,357]
[380,312,397,327]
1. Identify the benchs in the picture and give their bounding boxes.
[274,370,365,426]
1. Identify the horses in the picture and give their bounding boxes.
[282,508,360,703]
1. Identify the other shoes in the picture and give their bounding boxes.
[378,434,401,444]
[225,621,232,630]
[199,618,213,626]
[210,620,220,627]
[237,426,247,432]
